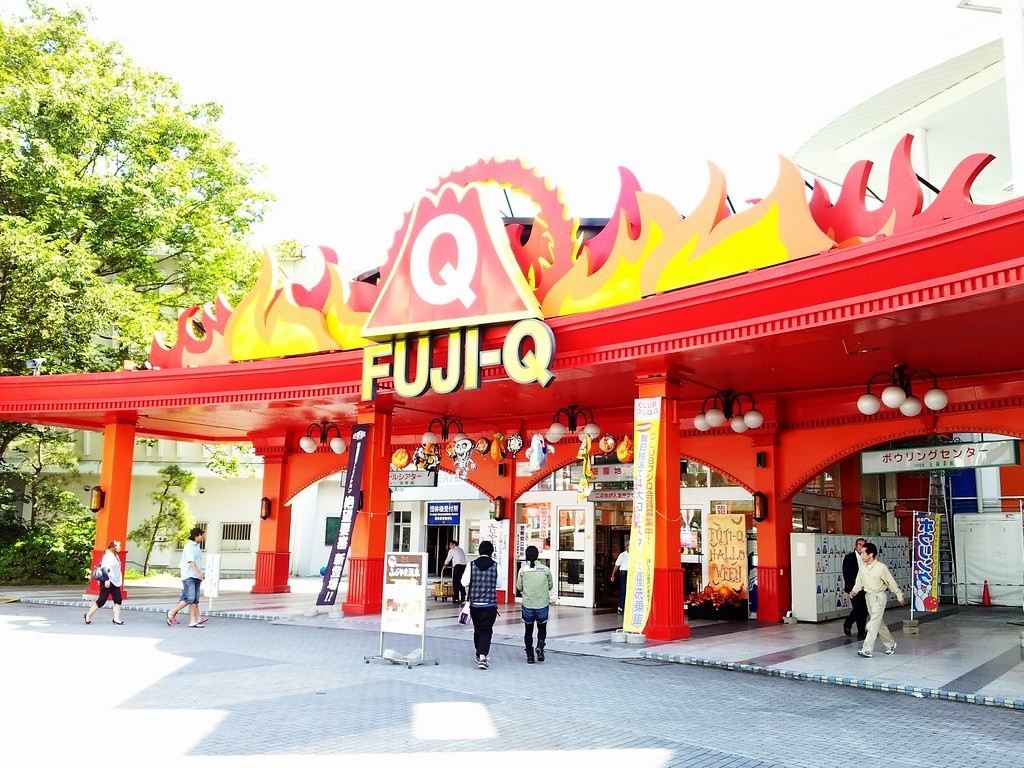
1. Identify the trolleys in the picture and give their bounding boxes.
[433,566,454,601]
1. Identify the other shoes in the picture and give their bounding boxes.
[197,617,208,624]
[475,657,480,662]
[170,617,180,624]
[478,660,489,668]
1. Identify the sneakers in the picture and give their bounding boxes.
[886,642,897,655]
[858,650,872,657]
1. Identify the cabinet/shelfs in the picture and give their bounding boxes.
[789,532,914,623]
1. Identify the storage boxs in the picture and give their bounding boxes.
[432,584,455,596]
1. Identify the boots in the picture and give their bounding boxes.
[536,640,546,661]
[524,647,534,663]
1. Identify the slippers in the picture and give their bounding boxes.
[189,623,205,628]
[167,610,172,626]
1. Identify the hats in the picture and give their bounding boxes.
[190,528,204,537]
[625,540,629,546]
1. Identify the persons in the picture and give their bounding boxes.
[842,539,868,641]
[850,543,905,658]
[516,546,554,662]
[611,540,629,614]
[444,540,467,604]
[166,528,209,628]
[83,540,125,625]
[460,541,503,669]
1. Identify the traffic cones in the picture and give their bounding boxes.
[978,580,994,608]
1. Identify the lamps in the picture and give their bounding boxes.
[299,420,346,455]
[692,388,764,434]
[387,487,393,516]
[259,496,271,521]
[856,362,948,416]
[89,486,106,513]
[493,493,506,522]
[421,416,468,449]
[545,405,601,443]
[752,490,770,523]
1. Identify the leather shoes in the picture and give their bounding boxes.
[843,623,851,637]
[857,632,867,641]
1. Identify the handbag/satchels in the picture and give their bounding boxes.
[458,601,472,625]
[93,564,108,581]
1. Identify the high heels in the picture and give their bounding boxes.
[84,613,92,624]
[113,619,124,625]
